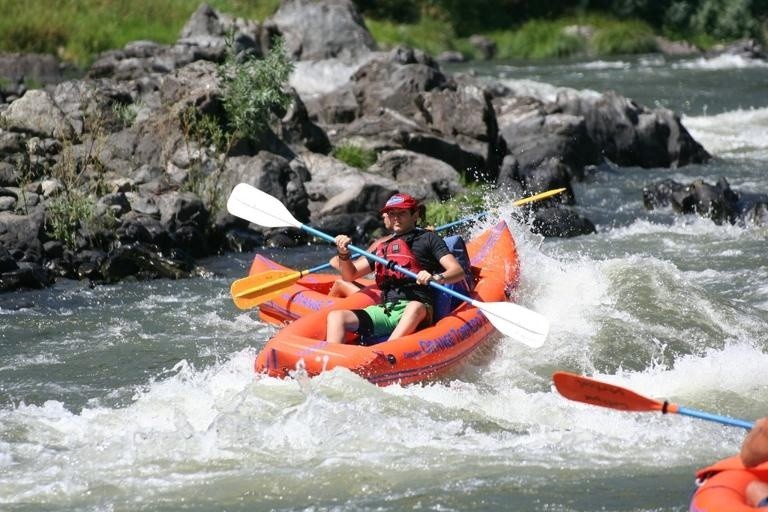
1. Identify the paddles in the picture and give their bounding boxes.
[226,183,550,349]
[553,371,755,430]
[231,187,566,310]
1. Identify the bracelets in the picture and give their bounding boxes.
[438,273,446,285]
[337,250,352,261]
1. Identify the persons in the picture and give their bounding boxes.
[329,199,434,298]
[740,416,768,506]
[327,195,465,344]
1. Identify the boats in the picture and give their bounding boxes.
[247,252,381,330]
[689,454,767,512]
[254,219,521,387]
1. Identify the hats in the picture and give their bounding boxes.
[381,194,417,212]
